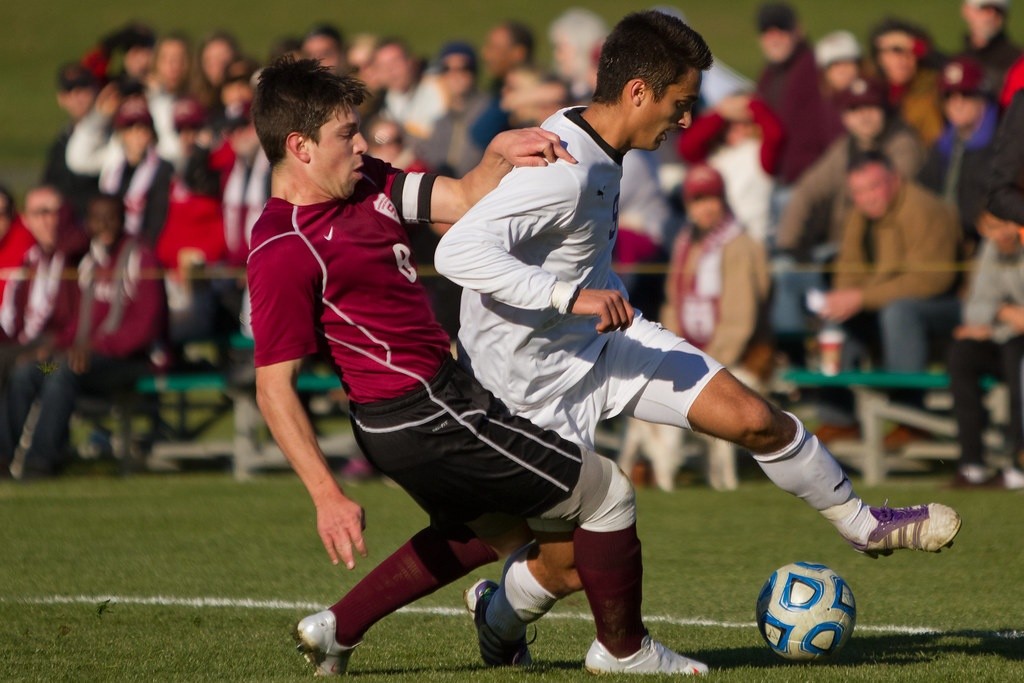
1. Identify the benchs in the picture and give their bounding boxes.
[129,332,348,480]
[782,364,1001,488]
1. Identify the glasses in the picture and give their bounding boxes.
[879,43,908,52]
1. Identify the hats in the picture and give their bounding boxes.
[841,77,885,107]
[936,58,980,95]
[815,28,863,69]
[225,99,254,131]
[218,57,260,85]
[175,99,205,126]
[434,40,477,75]
[115,97,153,128]
[684,165,725,203]
[57,60,92,91]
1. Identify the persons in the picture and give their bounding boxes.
[436,10,960,672]
[246,57,711,675]
[0,0,1024,490]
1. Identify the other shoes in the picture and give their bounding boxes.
[1003,468,1024,490]
[952,465,986,486]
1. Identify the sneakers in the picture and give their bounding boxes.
[850,498,962,560]
[463,577,538,668]
[291,609,364,678]
[584,636,708,674]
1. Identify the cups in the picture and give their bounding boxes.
[816,327,847,377]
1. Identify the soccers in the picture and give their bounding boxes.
[755,560,856,660]
